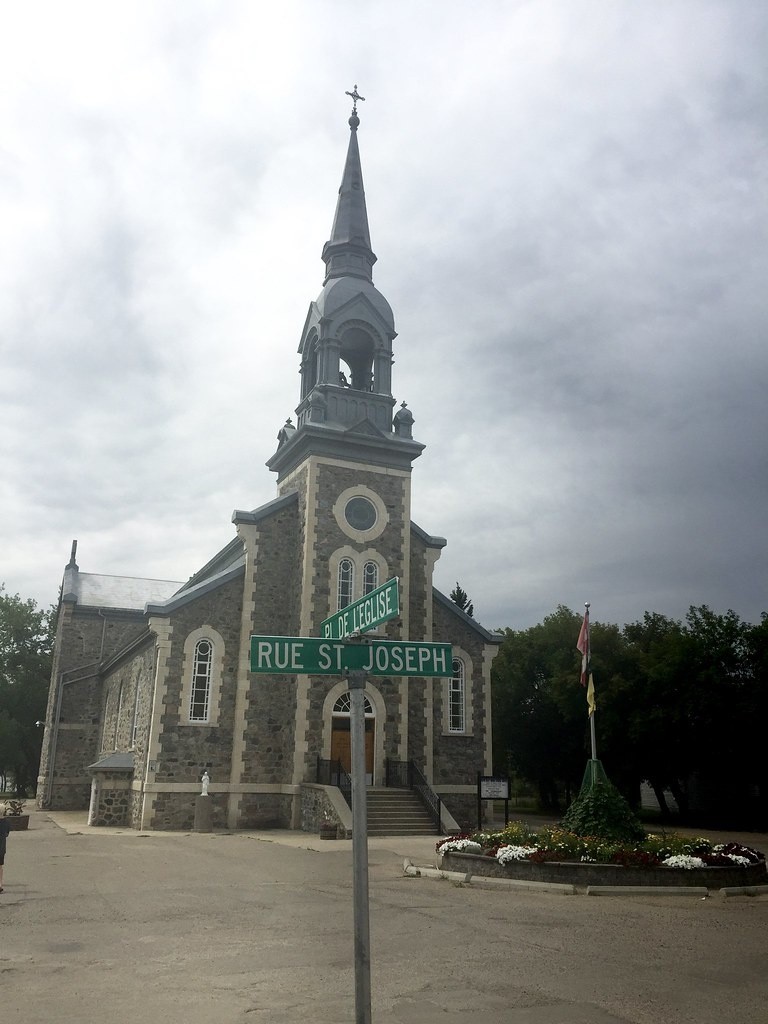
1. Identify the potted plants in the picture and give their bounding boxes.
[3,799,30,831]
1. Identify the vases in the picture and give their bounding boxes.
[320,828,338,839]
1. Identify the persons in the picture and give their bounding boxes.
[201,772,209,795]
[0,804,10,892]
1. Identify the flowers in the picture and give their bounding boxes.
[320,810,337,831]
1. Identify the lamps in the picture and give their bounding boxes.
[36,720,50,729]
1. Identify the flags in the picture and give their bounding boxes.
[587,673,596,719]
[577,611,589,686]
